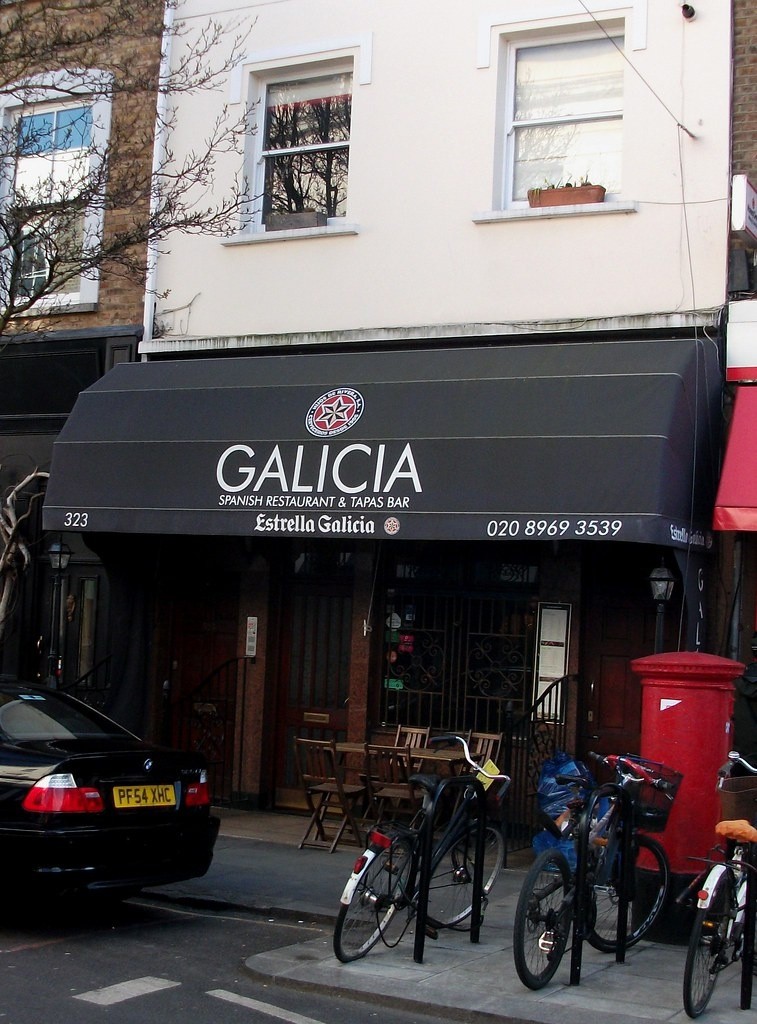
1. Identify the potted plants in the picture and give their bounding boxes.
[265,204,327,233]
[526,170,606,208]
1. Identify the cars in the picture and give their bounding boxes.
[0,672,221,925]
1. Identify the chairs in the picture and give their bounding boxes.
[363,743,427,844]
[293,735,366,853]
[434,728,505,835]
[360,724,431,831]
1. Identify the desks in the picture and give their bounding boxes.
[309,742,486,849]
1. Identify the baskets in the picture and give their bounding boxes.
[617,757,683,833]
[717,777,757,832]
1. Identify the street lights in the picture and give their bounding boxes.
[41,535,76,693]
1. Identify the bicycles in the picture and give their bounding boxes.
[511,750,683,990]
[331,733,511,964]
[680,751,756,1020]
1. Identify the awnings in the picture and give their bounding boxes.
[39,333,729,561]
